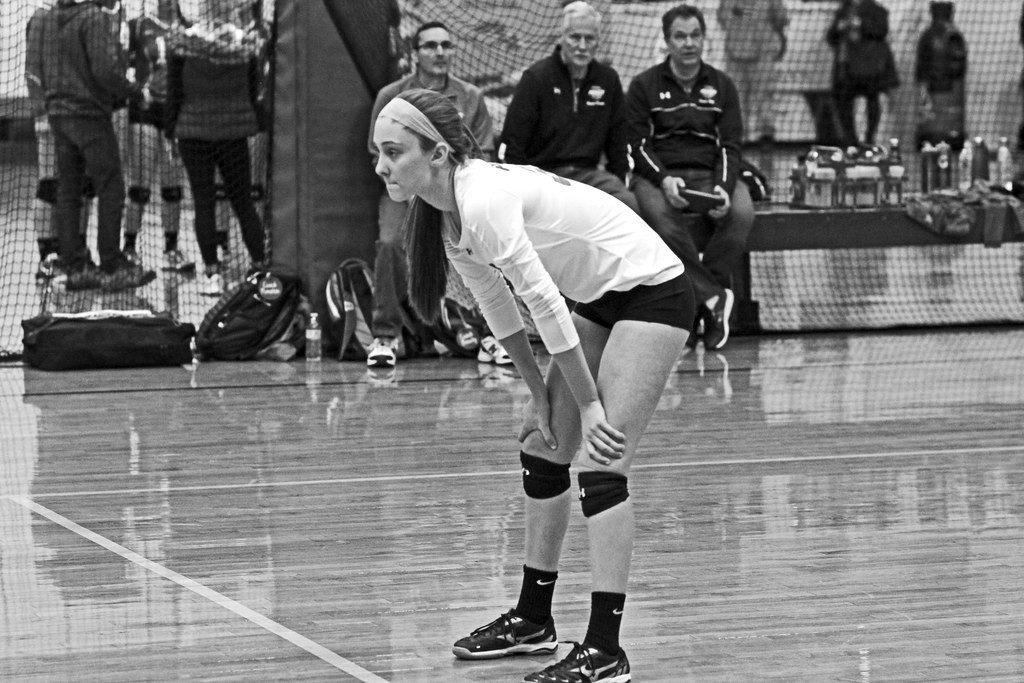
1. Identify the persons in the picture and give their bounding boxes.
[824,0,890,144]
[497,1,638,214]
[373,88,696,683]
[621,4,753,351]
[25,0,274,294]
[717,0,793,151]
[367,21,515,367]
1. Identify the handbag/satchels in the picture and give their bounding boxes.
[20,310,197,369]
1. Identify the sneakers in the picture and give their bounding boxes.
[451,608,558,659]
[521,640,631,683]
[475,335,513,365]
[365,336,398,366]
[686,288,734,351]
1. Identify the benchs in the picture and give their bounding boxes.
[681,192,1023,330]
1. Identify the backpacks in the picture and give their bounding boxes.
[197,267,301,361]
[326,256,421,362]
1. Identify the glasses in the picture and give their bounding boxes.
[415,42,456,54]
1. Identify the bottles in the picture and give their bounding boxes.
[922,135,990,194]
[995,136,1014,190]
[305,313,322,362]
[886,138,903,205]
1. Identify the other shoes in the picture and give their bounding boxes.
[65,263,99,292]
[35,254,67,285]
[100,263,158,293]
[161,249,196,272]
[198,270,225,295]
[124,250,144,267]
